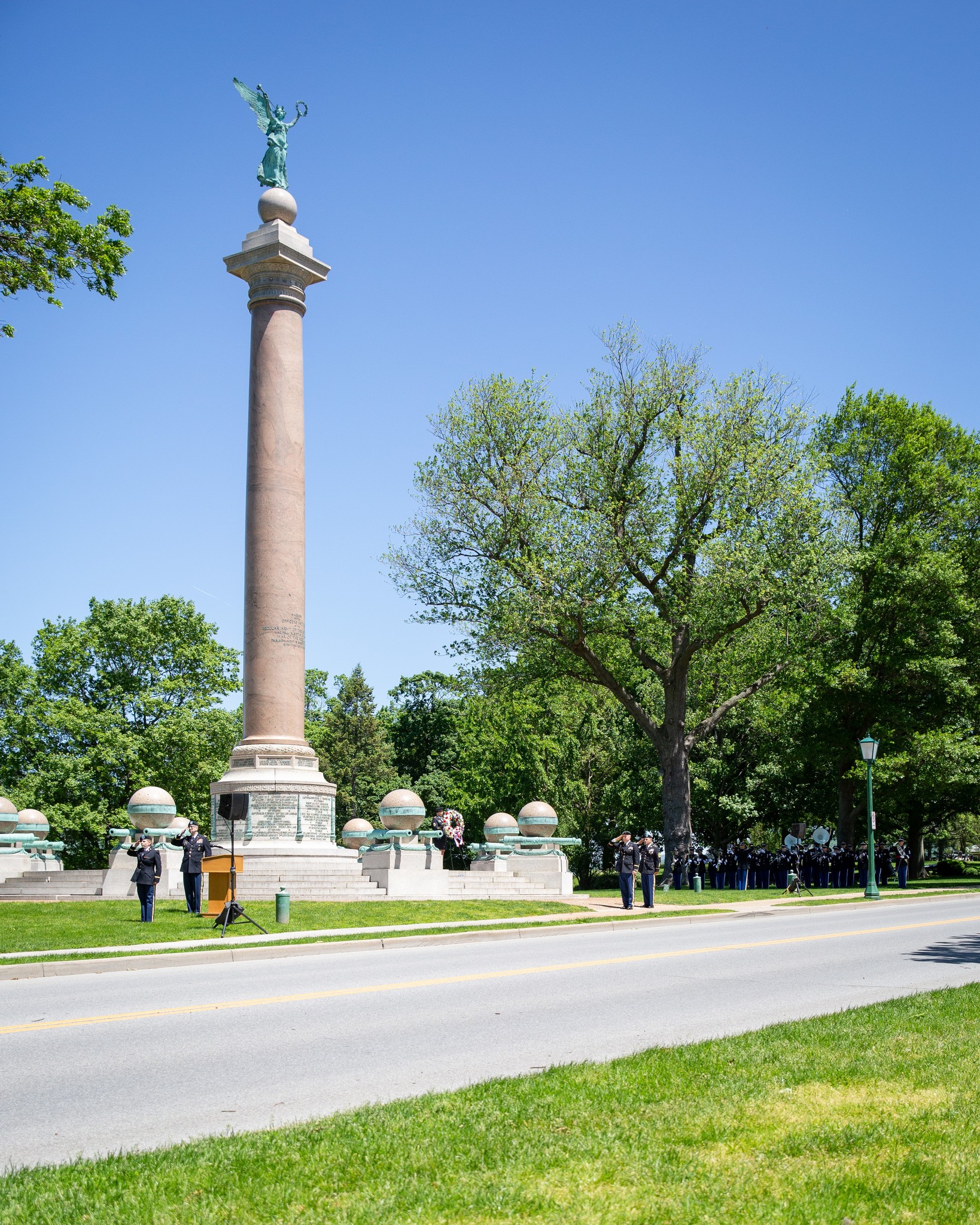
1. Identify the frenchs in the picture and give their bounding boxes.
[784,834,801,853]
[813,828,830,848]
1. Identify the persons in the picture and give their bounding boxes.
[432,807,447,859]
[697,847,708,890]
[831,841,856,888]
[127,835,161,923]
[872,843,883,886]
[737,837,756,890]
[670,847,683,890]
[855,841,868,889]
[708,847,718,888]
[607,831,640,909]
[879,842,892,885]
[725,845,738,889]
[712,848,728,890]
[747,842,822,890]
[171,821,212,913]
[816,844,832,888]
[684,847,700,890]
[636,834,661,908]
[890,839,911,889]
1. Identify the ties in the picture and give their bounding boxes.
[646,846,648,849]
[625,843,626,847]
[192,837,194,841]
[621,831,631,835]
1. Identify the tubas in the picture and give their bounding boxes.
[706,847,715,865]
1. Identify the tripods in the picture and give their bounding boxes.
[782,838,813,897]
[212,819,268,937]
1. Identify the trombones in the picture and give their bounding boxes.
[802,844,811,852]
[770,856,777,864]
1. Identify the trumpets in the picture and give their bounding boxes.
[757,848,765,853]
[727,848,731,851]
[791,850,797,854]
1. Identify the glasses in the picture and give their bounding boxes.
[188,826,196,829]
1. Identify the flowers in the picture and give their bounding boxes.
[444,809,465,839]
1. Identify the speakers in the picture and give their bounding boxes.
[217,793,249,820]
[790,823,806,839]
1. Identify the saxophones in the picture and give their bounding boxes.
[716,855,721,871]
[686,853,691,873]
[671,853,676,870]
[697,853,701,870]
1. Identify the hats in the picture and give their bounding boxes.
[732,845,735,848]
[689,846,724,852]
[673,847,679,851]
[436,807,444,813]
[643,834,652,838]
[775,843,789,852]
[140,835,152,841]
[795,843,809,847]
[739,842,746,847]
[188,821,198,827]
[761,843,768,847]
[822,837,905,849]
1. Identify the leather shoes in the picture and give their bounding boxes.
[640,905,654,908]
[620,906,633,910]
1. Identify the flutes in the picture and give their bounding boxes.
[832,851,836,857]
[840,847,843,858]
[860,847,864,862]
[819,850,824,865]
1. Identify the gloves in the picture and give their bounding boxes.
[841,848,844,852]
[900,855,904,858]
[727,837,765,853]
[708,858,712,862]
[893,842,898,846]
[674,859,677,863]
[832,853,837,856]
[716,860,722,869]
[688,859,692,863]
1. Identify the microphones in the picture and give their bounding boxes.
[210,844,232,853]
